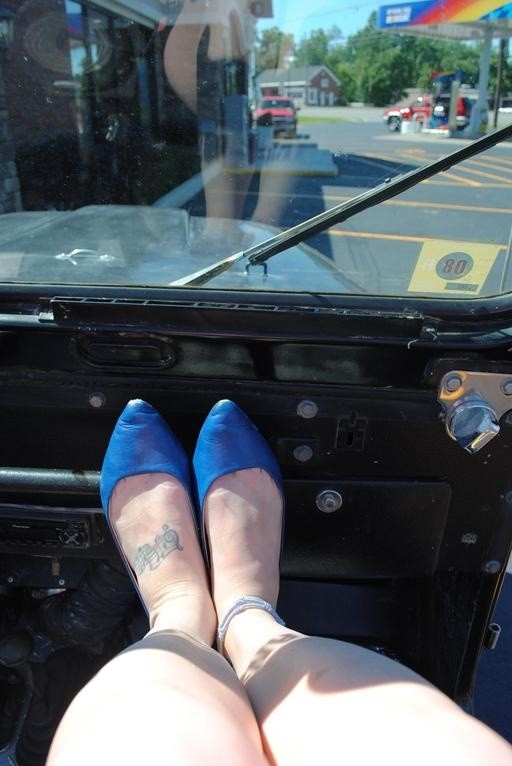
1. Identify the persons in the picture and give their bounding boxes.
[161,0,326,262]
[41,396,512,765]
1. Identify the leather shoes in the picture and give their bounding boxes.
[95,395,205,623]
[189,397,287,562]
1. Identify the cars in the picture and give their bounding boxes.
[383,93,472,132]
[252,96,296,137]
